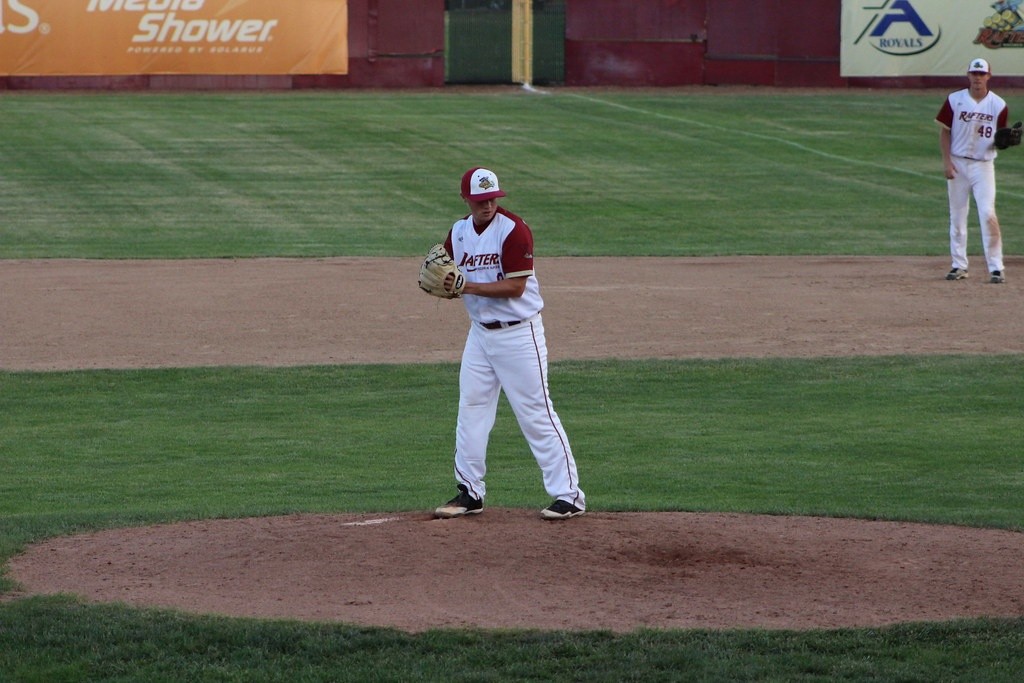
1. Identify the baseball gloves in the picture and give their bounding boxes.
[994,121,1024,150]
[416,242,467,300]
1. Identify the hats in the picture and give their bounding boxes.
[461,167,505,201]
[968,58,991,73]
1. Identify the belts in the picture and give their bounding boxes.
[480,310,540,329]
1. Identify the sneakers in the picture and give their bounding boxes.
[540,500,584,517]
[946,268,969,279]
[435,484,484,515]
[991,271,1005,282]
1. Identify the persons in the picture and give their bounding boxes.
[416,166,586,521]
[932,56,1024,284]
